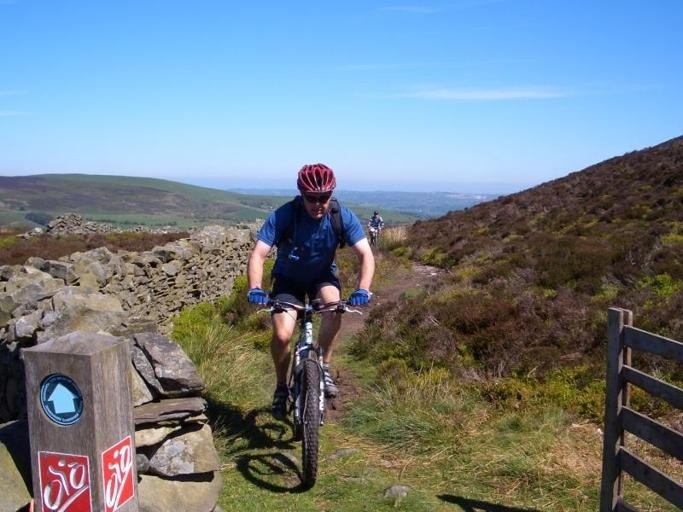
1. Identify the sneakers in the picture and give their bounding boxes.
[270,383,290,420]
[320,368,338,399]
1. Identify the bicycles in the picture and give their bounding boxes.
[369,225,385,247]
[252,295,366,489]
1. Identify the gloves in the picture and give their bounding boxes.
[348,288,369,306]
[247,287,269,305]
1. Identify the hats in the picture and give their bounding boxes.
[296,162,337,195]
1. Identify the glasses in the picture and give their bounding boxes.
[302,193,329,204]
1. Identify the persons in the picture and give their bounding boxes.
[247,162,375,420]
[367,209,385,245]
